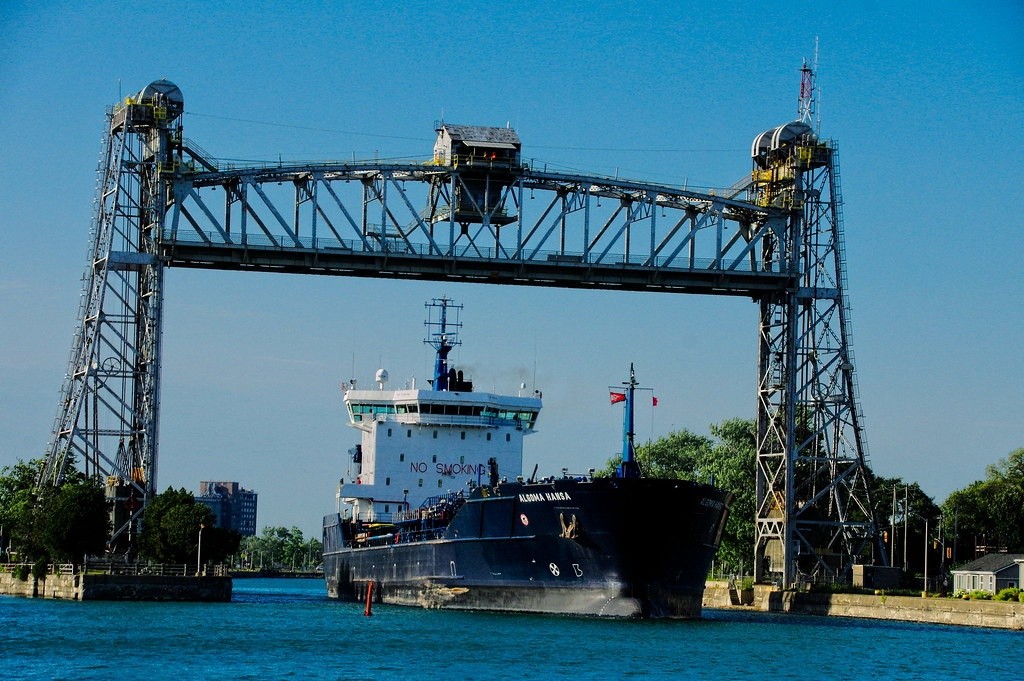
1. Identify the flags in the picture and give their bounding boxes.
[653,397,657,406]
[609,392,625,403]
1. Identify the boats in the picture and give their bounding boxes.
[318,291,736,622]
